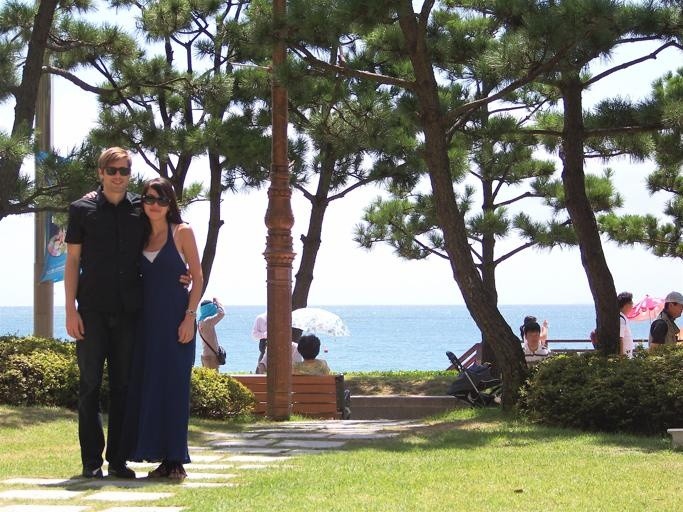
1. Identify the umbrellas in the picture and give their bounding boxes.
[626,293,665,323]
[291,307,351,337]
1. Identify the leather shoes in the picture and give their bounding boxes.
[108,461,135,480]
[83,466,103,481]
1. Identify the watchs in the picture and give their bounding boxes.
[186,310,197,317]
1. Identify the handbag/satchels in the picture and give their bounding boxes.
[217,346,227,365]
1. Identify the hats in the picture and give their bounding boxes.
[665,291,683,305]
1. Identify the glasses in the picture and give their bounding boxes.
[142,194,171,207]
[104,167,131,177]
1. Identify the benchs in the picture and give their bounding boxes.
[229,374,351,420]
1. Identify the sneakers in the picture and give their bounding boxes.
[148,461,188,482]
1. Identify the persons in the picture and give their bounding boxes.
[617,292,633,358]
[81,177,204,482]
[63,146,193,481]
[519,315,548,347]
[648,291,683,350]
[252,310,331,375]
[196,297,225,374]
[521,321,551,369]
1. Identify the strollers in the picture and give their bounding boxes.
[448,351,502,407]
